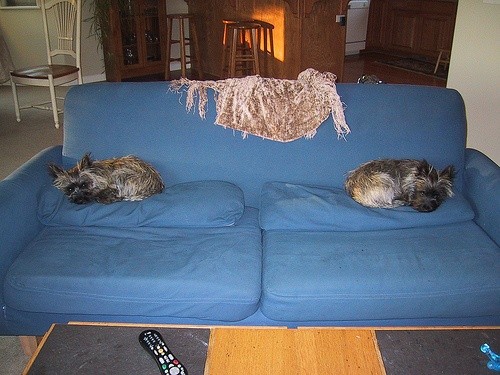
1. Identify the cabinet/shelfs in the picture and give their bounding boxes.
[98,0,168,82]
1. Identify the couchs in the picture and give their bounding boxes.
[0,81,500,355]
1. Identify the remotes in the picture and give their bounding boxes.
[138,330,188,375]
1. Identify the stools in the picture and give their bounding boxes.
[434,48,451,75]
[222,18,261,79]
[165,14,203,81]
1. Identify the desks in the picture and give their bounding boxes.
[20,323,500,374]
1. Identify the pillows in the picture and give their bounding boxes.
[38,180,245,228]
[258,182,475,230]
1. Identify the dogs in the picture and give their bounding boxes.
[344,158,455,213]
[48,152,164,204]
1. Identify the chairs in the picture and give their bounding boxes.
[8,0,83,129]
